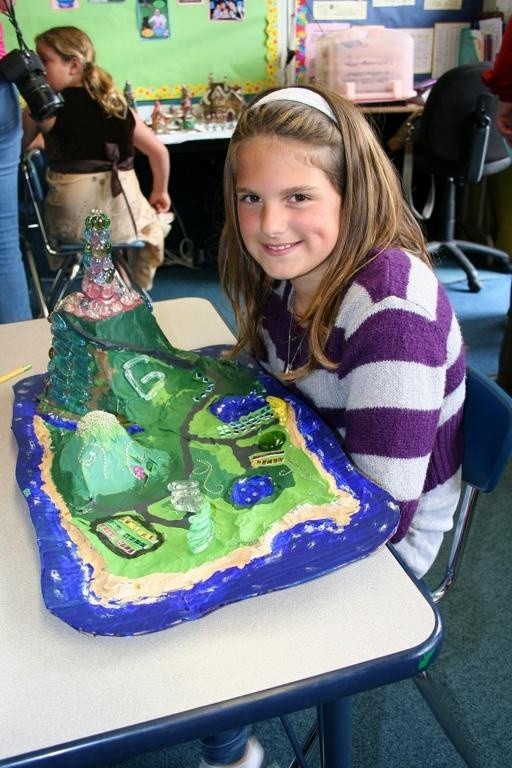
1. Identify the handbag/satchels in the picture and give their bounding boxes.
[388,106,424,152]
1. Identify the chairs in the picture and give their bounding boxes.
[405,63,511,293]
[20,146,156,319]
[277,367,511,768]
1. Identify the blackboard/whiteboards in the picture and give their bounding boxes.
[0,0,289,106]
[289,1,512,100]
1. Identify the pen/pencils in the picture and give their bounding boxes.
[0,362,33,383]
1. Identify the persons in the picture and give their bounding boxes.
[23,27,170,294]
[212,1,243,18]
[199,83,468,768]
[0,0,32,324]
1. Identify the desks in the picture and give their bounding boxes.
[359,101,511,267]
[145,121,230,270]
[0,295,444,768]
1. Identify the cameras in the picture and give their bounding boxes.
[0,49,65,121]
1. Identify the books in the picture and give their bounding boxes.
[431,16,502,78]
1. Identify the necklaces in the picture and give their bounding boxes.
[284,296,310,375]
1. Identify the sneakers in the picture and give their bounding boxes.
[197,735,266,768]
